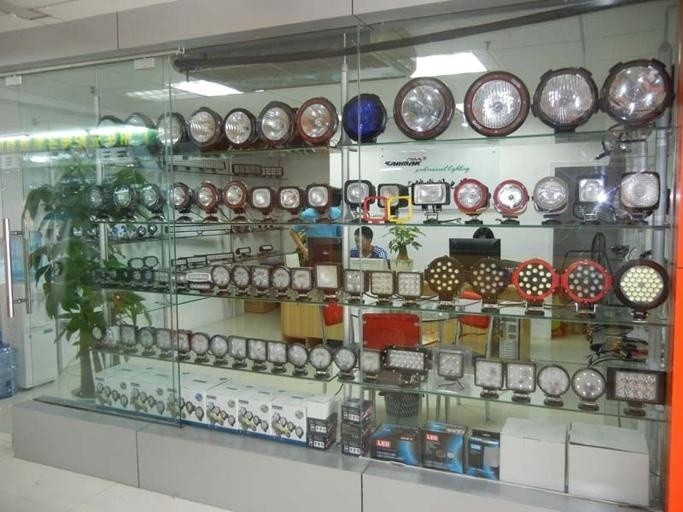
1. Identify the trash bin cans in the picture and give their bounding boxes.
[386,391,421,416]
[0,345,15,398]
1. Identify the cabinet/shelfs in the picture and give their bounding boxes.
[9,0,683,512]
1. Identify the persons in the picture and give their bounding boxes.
[288,182,343,265]
[472,226,494,239]
[350,226,387,258]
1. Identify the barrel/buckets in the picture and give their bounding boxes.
[9,231,42,282]
[0,331,15,399]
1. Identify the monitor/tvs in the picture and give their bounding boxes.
[307,237,342,263]
[449,237,501,261]
